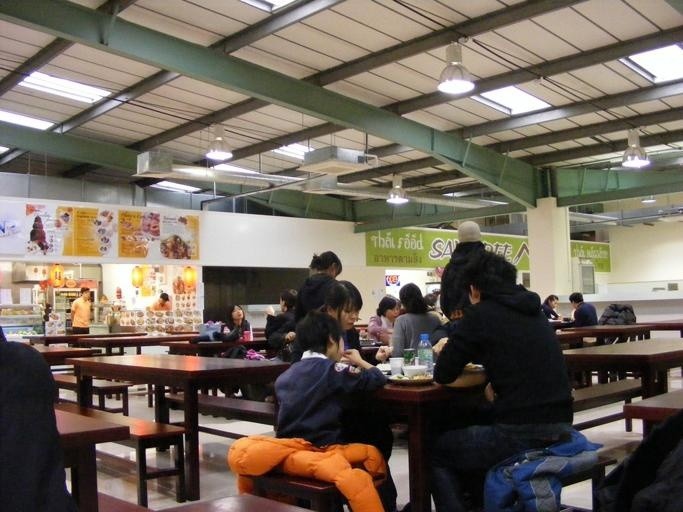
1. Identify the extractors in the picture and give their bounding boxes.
[11,263,49,283]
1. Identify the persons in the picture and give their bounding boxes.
[153,293,171,311]
[0,324,83,512]
[70,287,92,376]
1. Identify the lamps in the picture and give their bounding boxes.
[434,38,475,96]
[203,122,233,161]
[49,263,64,286]
[181,265,196,289]
[385,171,409,205]
[618,127,650,168]
[131,266,143,288]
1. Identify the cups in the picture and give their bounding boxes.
[388,357,405,376]
[244,331,251,341]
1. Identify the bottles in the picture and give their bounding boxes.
[417,333,434,378]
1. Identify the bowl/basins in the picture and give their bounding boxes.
[401,364,427,378]
[360,339,374,345]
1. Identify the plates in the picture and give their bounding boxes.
[387,374,434,386]
[465,363,484,369]
[375,363,392,373]
[94,219,113,254]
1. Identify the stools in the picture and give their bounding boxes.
[239,472,386,511]
[555,454,618,511]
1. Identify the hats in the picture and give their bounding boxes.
[459,220,480,243]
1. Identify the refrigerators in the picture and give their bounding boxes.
[47,288,99,334]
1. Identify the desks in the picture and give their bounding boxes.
[356,325,487,511]
[21,327,291,511]
[549,318,682,435]
